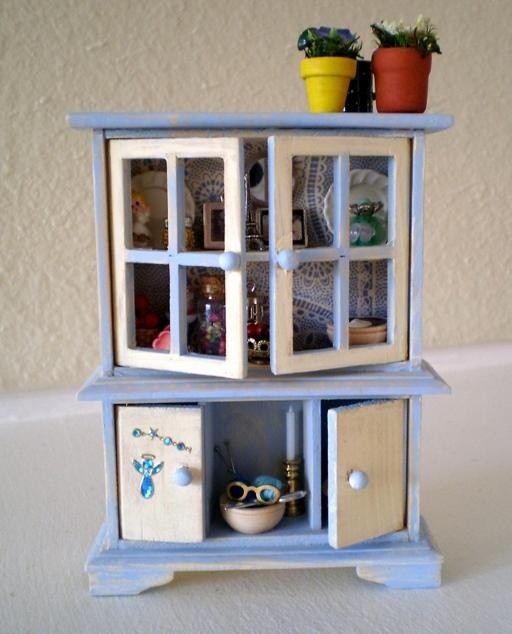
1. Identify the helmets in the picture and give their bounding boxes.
[131,169,198,251]
[322,165,388,246]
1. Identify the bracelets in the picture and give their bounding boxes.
[324,315,388,347]
[219,490,287,534]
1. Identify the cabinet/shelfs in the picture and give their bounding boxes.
[195,280,225,357]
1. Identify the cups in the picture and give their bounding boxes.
[227,481,281,505]
